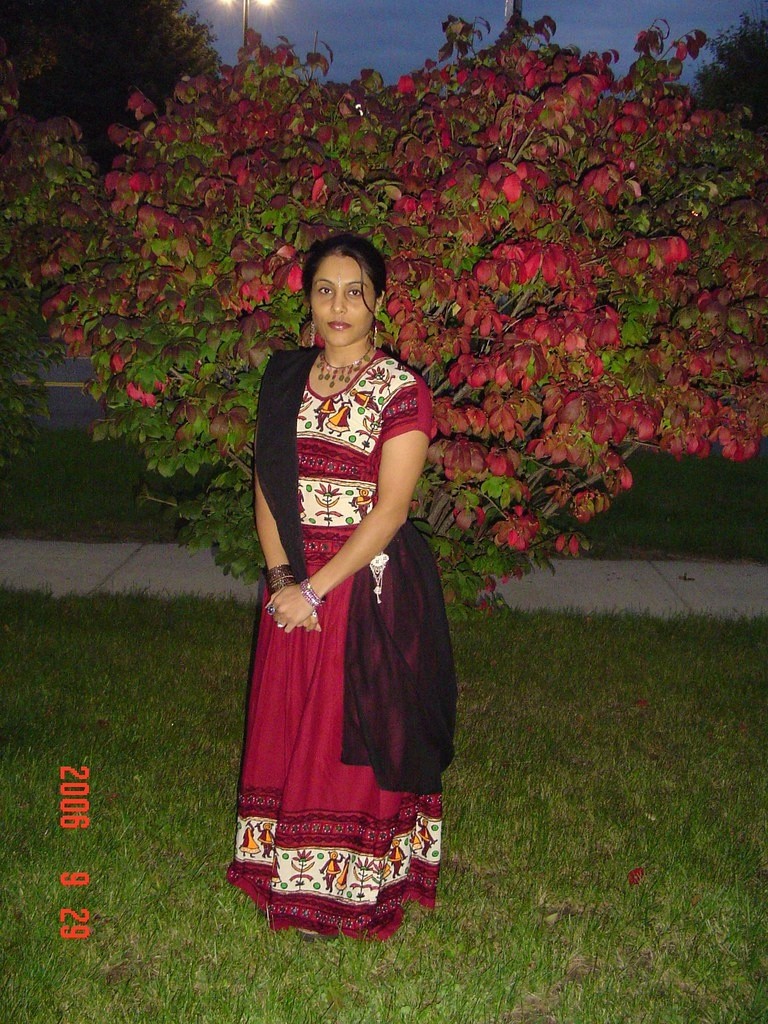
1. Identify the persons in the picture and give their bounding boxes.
[253,233,441,944]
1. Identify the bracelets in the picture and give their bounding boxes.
[300,578,327,618]
[266,564,297,595]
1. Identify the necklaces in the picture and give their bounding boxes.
[315,344,373,389]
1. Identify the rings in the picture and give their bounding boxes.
[266,603,276,615]
[277,620,285,628]
